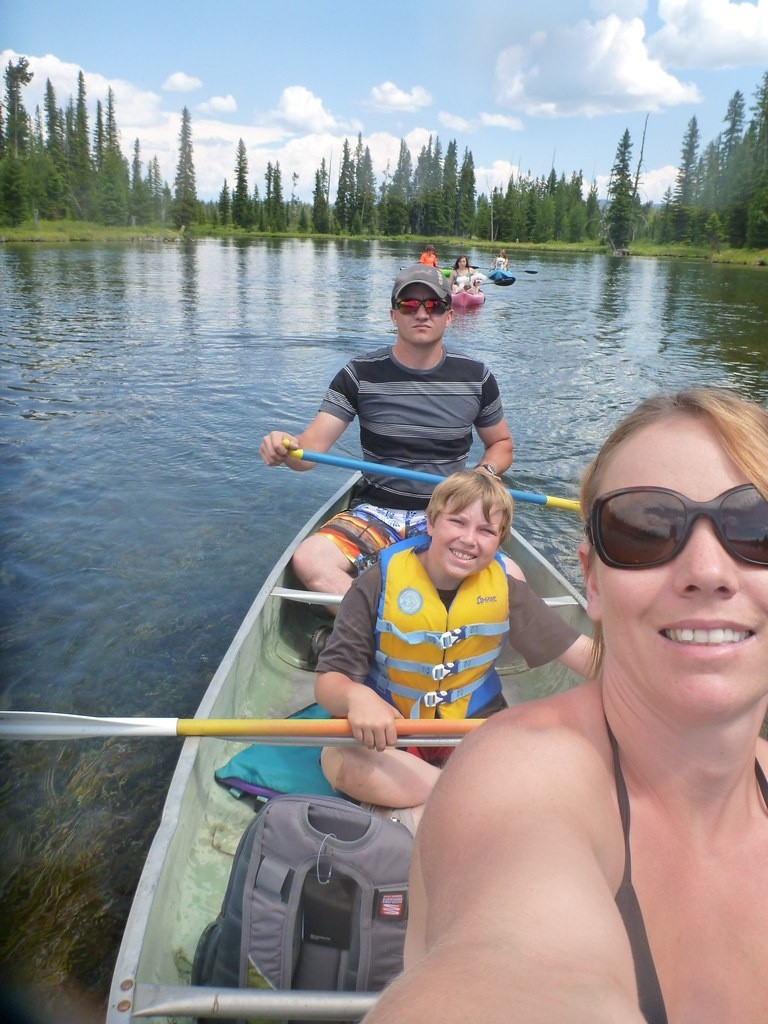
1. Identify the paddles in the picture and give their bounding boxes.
[480,278,515,285]
[468,266,538,274]
[0,709,493,747]
[283,438,581,513]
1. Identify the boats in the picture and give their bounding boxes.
[106,451,590,1024]
[436,265,454,278]
[448,285,488,307]
[487,269,516,285]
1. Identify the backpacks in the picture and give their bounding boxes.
[193,793,416,1024]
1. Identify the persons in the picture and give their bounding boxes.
[490,250,508,270]
[257,264,528,665]
[311,470,605,809]
[356,388,768,1023]
[448,256,479,295]
[419,244,440,272]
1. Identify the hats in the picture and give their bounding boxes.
[391,264,453,305]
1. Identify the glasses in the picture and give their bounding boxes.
[394,298,450,314]
[587,482,767,569]
